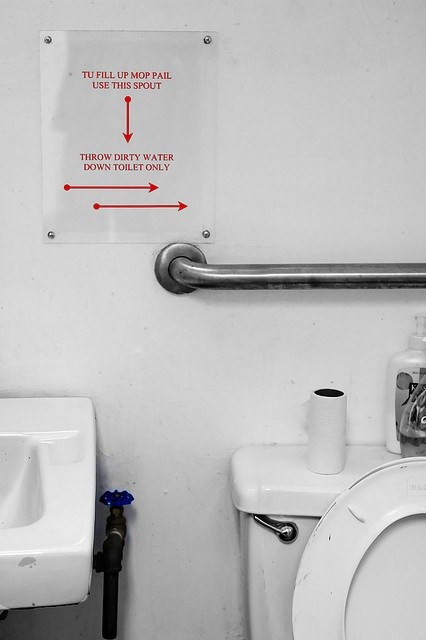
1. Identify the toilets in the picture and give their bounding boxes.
[228,443,426,640]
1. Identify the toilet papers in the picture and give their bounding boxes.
[306,388,348,475]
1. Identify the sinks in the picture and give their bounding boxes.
[0,396,96,612]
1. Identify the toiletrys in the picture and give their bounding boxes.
[383,313,426,457]
